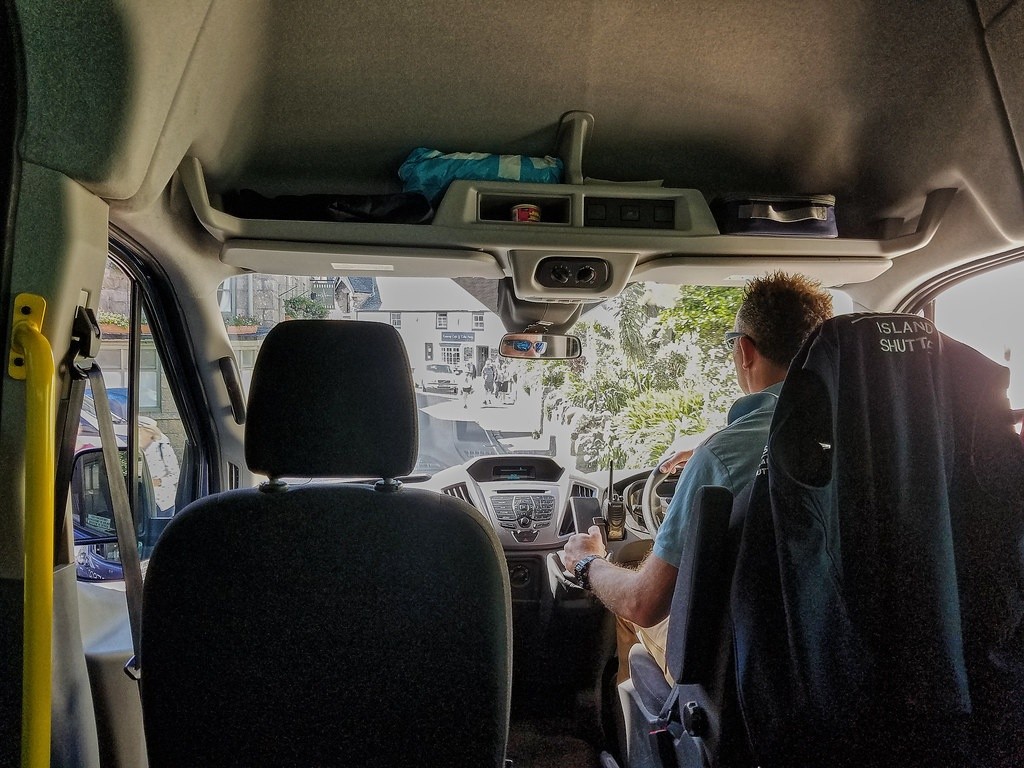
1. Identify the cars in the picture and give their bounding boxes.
[413,362,460,395]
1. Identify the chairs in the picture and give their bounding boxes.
[623,311,1024,768]
[137,319,515,768]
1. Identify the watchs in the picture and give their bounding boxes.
[574,555,609,592]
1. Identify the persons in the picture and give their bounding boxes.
[500,335,547,358]
[563,269,836,689]
[456,358,511,409]
[137,415,181,516]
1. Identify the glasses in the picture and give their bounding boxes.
[502,338,548,355]
[724,331,756,350]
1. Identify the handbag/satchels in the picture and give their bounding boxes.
[709,191,841,239]
